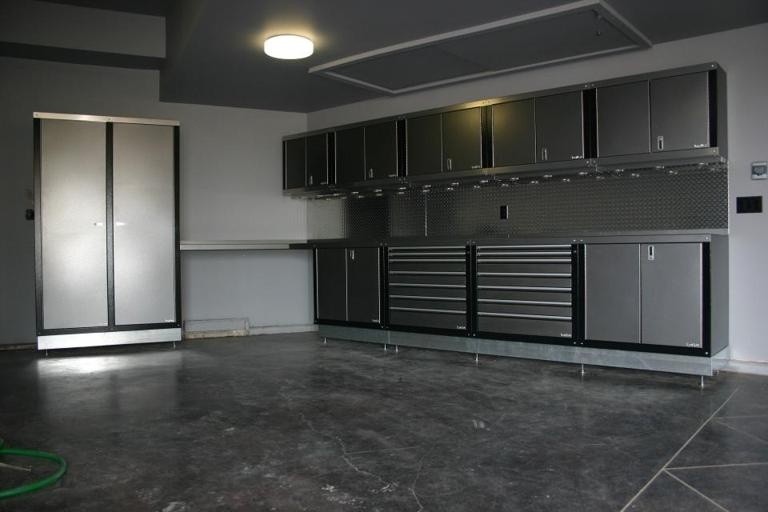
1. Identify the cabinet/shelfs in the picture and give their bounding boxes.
[313,247,386,351]
[580,243,729,376]
[595,62,727,172]
[330,114,409,191]
[282,126,337,195]
[33,111,182,356]
[401,99,490,189]
[484,82,596,178]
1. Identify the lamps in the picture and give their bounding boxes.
[263,34,314,60]
